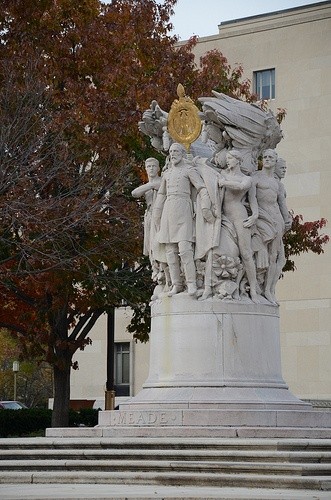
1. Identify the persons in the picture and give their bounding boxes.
[154,142,216,297]
[131,157,165,282]
[197,150,261,304]
[232,147,286,307]
[270,155,286,305]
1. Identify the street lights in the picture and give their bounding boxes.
[12,361,19,401]
